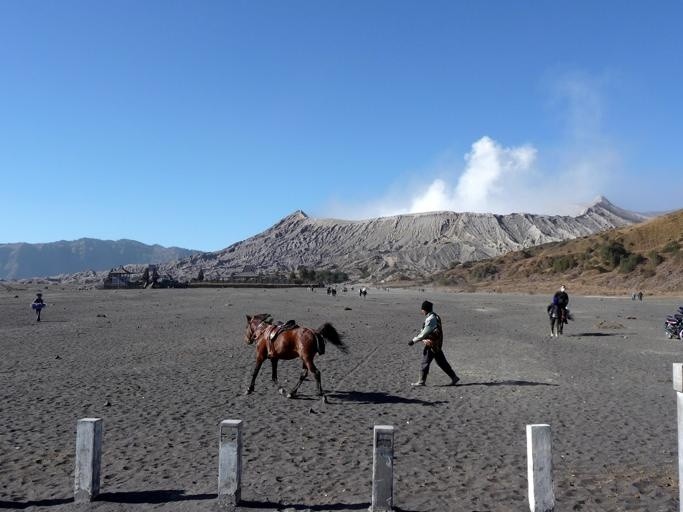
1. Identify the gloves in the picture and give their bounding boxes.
[408,340,414,347]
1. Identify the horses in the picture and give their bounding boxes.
[547,303,575,339]
[243,313,349,398]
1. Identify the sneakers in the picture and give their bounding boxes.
[410,383,425,386]
[450,376,460,386]
[563,319,568,324]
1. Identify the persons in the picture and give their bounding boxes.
[327,285,336,297]
[552,285,569,324]
[33,293,43,321]
[407,300,460,387]
[359,287,368,298]
[636,291,643,303]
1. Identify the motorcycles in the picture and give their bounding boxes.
[665,306,683,340]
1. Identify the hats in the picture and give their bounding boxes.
[421,301,433,312]
